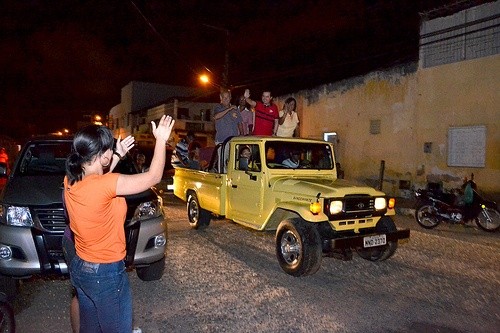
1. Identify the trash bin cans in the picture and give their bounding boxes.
[427,181,443,193]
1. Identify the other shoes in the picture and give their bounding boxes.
[132,327,142,333]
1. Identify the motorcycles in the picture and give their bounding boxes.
[412,173,500,232]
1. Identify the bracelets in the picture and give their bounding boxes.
[114,149,124,161]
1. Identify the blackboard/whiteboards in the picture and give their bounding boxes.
[447,125,486,168]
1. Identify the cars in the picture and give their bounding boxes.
[0,138,168,282]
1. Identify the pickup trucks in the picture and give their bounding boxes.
[172,124,410,277]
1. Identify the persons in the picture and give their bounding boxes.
[171,131,214,169]
[212,87,300,146]
[62,115,175,333]
[236,147,260,172]
[282,149,302,169]
